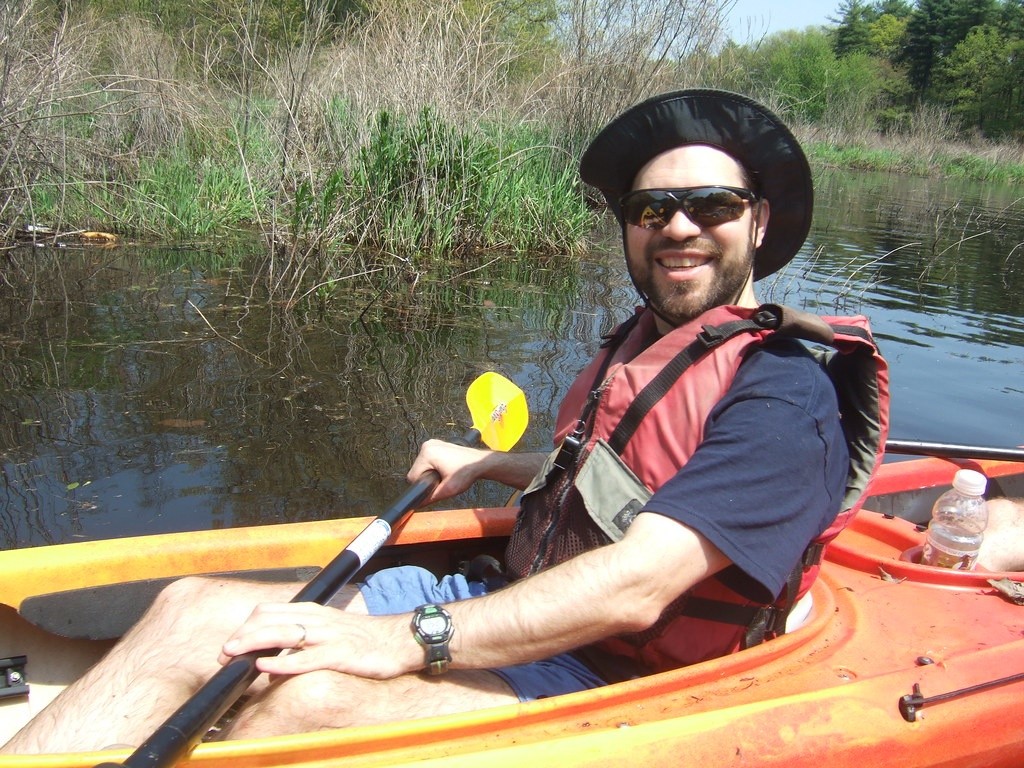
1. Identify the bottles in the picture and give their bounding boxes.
[919,469,987,572]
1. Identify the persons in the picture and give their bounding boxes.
[0,87,890,768]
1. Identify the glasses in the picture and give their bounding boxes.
[619,185,758,230]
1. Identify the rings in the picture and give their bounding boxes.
[290,622,307,650]
[243,618,255,635]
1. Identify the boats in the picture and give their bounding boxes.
[1,442,1024,768]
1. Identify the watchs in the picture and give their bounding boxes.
[410,602,456,678]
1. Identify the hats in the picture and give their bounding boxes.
[580,89,814,282]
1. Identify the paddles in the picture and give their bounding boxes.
[91,372,529,768]
